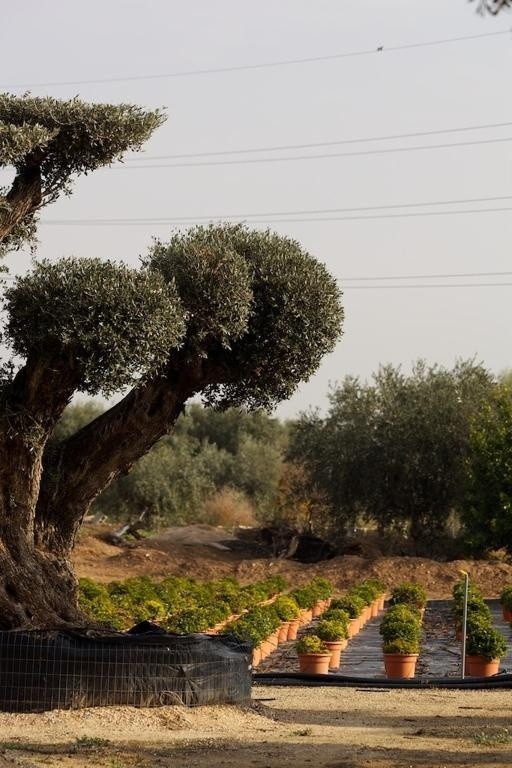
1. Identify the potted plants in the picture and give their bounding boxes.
[160,575,388,674]
[379,583,429,678]
[452,582,512,678]
